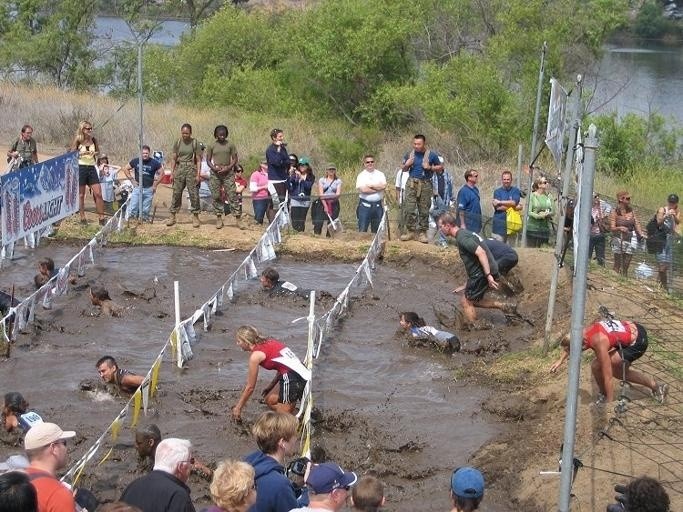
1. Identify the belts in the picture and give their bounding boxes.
[362,202,380,208]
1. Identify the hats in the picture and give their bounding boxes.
[306,463,358,495]
[451,467,484,498]
[199,143,206,149]
[326,162,336,170]
[24,423,76,450]
[297,158,309,167]
[667,194,679,202]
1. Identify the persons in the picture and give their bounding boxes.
[451,231,525,296]
[210,411,386,510]
[206,125,246,230]
[120,423,212,511]
[167,124,202,226]
[4,124,39,171]
[33,273,48,290]
[97,154,120,214]
[314,162,342,237]
[357,155,387,233]
[37,257,98,290]
[124,145,165,220]
[395,134,454,242]
[0,391,97,511]
[435,211,517,325]
[250,128,315,231]
[524,171,682,299]
[455,168,482,234]
[69,119,108,226]
[396,311,461,354]
[490,170,523,246]
[77,355,146,396]
[259,265,338,307]
[200,141,247,216]
[231,323,311,426]
[559,315,671,408]
[89,285,130,318]
[606,474,670,512]
[450,467,483,510]
[0,290,65,333]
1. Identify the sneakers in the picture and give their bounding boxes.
[81,219,88,225]
[417,233,428,243]
[99,218,107,226]
[656,383,669,404]
[504,303,517,327]
[400,230,415,240]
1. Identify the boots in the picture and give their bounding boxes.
[236,215,245,230]
[167,212,176,226]
[192,213,200,228]
[216,215,224,229]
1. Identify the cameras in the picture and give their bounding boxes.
[280,143,288,148]
[288,168,296,175]
[291,457,311,476]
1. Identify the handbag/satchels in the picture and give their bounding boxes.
[597,216,610,233]
[646,219,667,254]
[506,207,523,235]
[311,199,323,225]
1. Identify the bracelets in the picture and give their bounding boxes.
[130,177,133,180]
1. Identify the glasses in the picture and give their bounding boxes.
[181,458,194,464]
[84,127,92,130]
[236,170,241,173]
[44,440,66,447]
